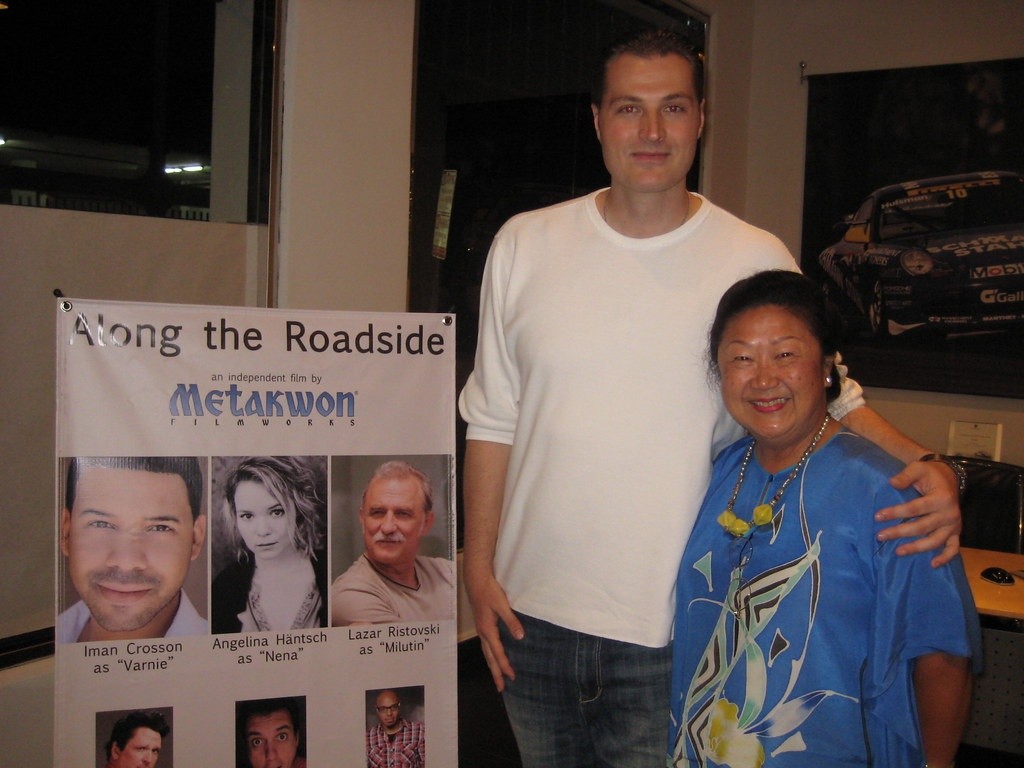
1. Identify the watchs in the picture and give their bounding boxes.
[913,450,970,496]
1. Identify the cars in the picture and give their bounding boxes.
[814,169,1024,351]
[948,452,1024,633]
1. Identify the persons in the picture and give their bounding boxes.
[454,22,965,768]
[105,684,425,768]
[57,453,455,654]
[664,269,977,768]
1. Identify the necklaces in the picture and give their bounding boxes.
[717,411,831,540]
